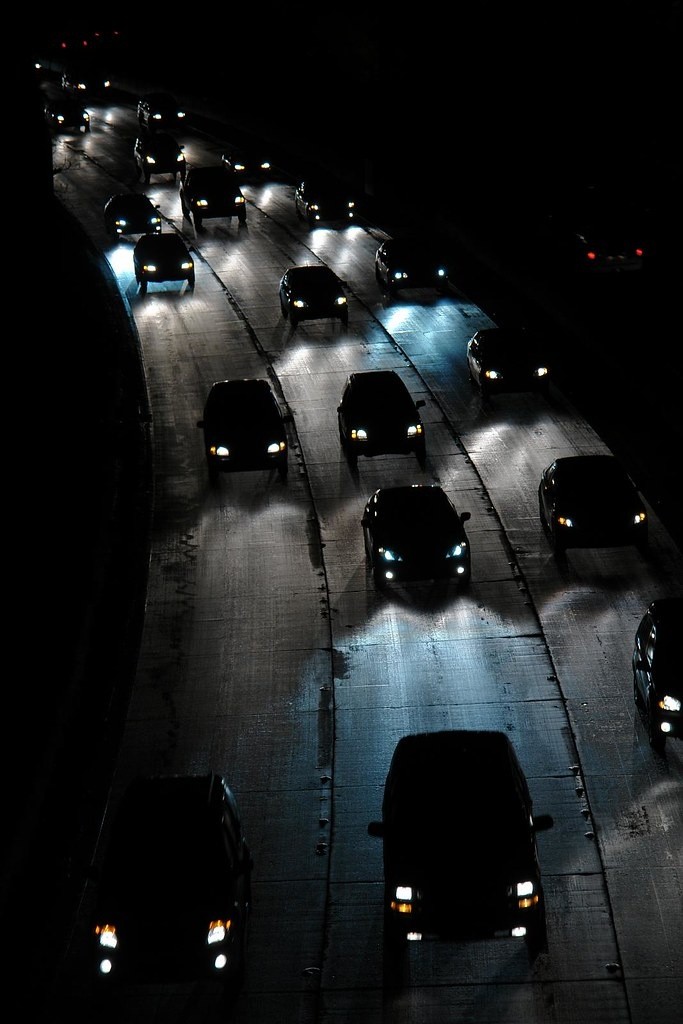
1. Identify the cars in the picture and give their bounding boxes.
[279,265,351,331]
[62,70,113,101]
[103,192,162,242]
[538,456,652,567]
[374,237,449,300]
[566,226,647,291]
[359,482,474,592]
[630,600,682,747]
[466,328,551,412]
[133,135,186,181]
[137,99,189,137]
[131,233,196,289]
[294,173,357,226]
[222,149,271,186]
[44,99,90,132]
[179,168,248,228]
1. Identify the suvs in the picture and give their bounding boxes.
[336,371,427,468]
[83,769,259,1005]
[194,380,296,483]
[366,728,550,957]
